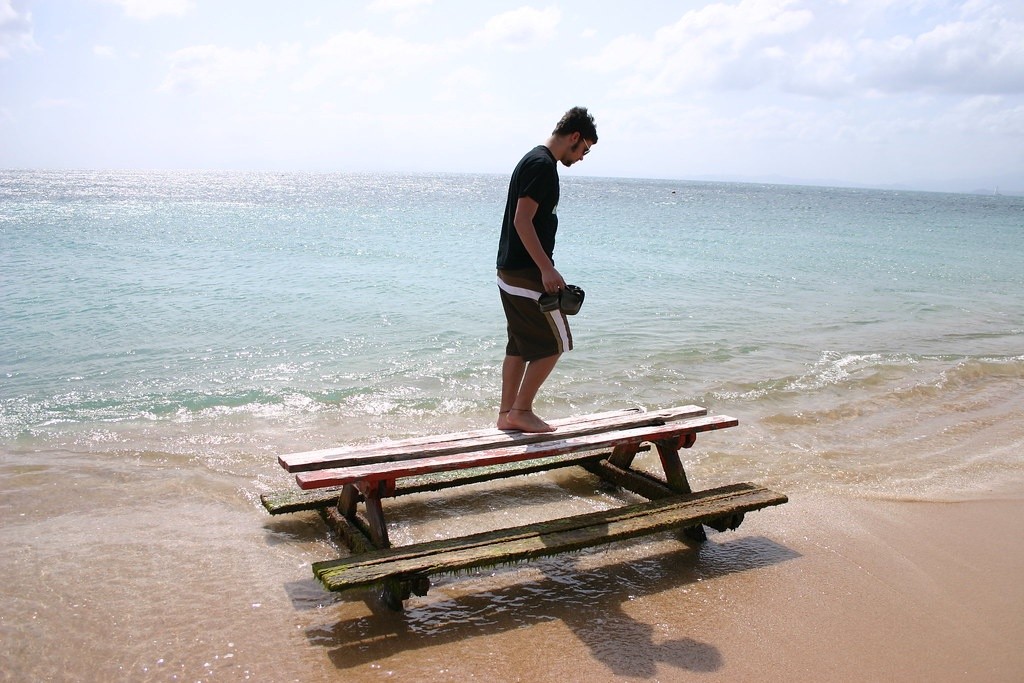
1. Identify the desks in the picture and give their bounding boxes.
[275,403,739,610]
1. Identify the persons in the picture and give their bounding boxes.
[495,107,598,431]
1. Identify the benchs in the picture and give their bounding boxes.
[310,480,789,609]
[261,442,651,515]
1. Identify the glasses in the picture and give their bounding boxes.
[583,138,591,155]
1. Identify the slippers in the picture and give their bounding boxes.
[538,291,559,312]
[559,283,585,315]
